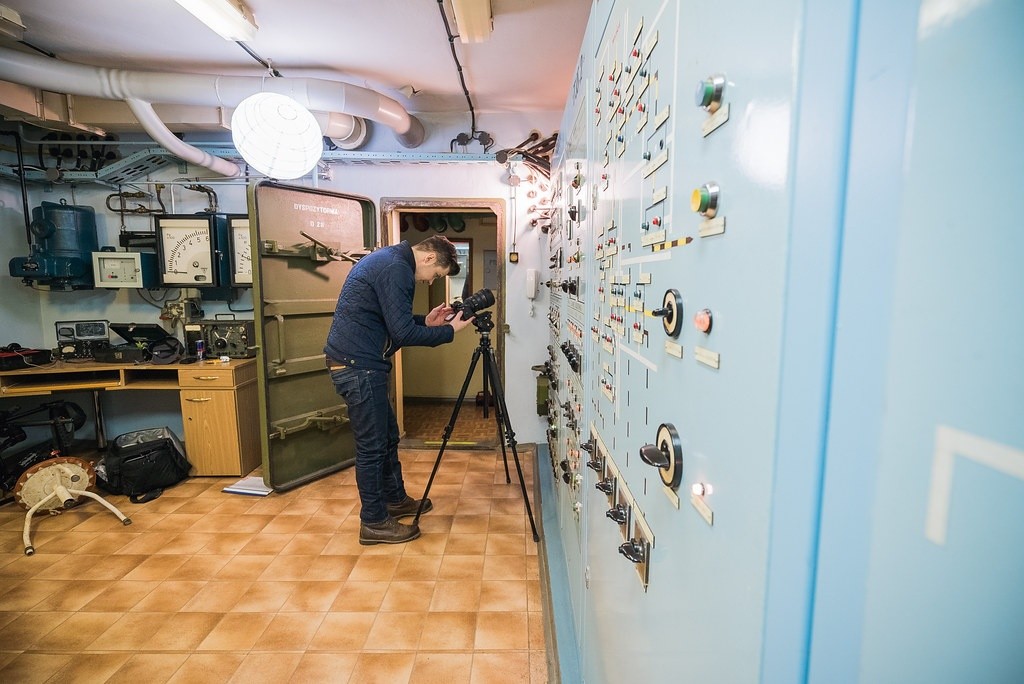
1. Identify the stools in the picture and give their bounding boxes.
[13,456,132,557]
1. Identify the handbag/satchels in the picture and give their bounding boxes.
[104,425,192,503]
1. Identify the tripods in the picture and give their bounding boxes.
[413,310,540,542]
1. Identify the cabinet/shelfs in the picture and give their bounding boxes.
[0,358,263,478]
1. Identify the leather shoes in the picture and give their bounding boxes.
[387,496,433,521]
[359,512,421,545]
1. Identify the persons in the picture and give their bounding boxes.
[323,234,476,545]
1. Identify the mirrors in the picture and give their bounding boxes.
[428,237,474,321]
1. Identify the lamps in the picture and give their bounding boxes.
[174,0,259,42]
[450,0,495,44]
[0,2,29,47]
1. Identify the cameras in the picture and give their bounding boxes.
[451,288,495,322]
